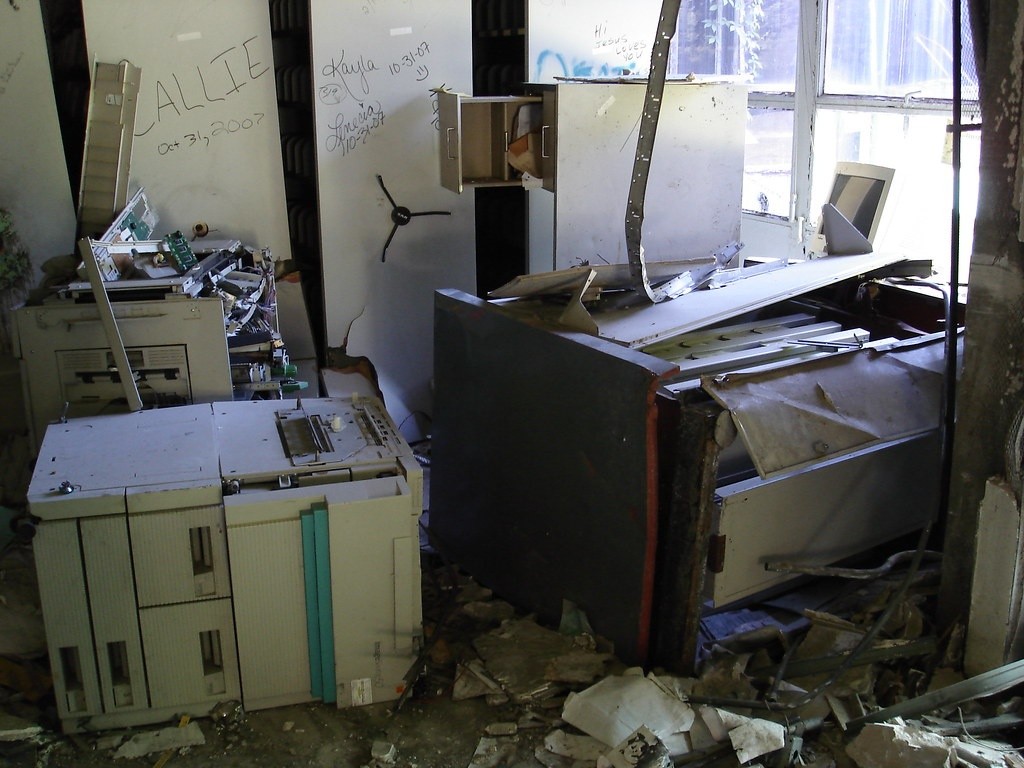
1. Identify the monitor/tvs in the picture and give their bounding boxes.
[812,163,896,252]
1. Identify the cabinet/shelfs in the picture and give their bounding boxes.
[435,80,750,270]
[24,392,425,729]
[433,282,966,678]
